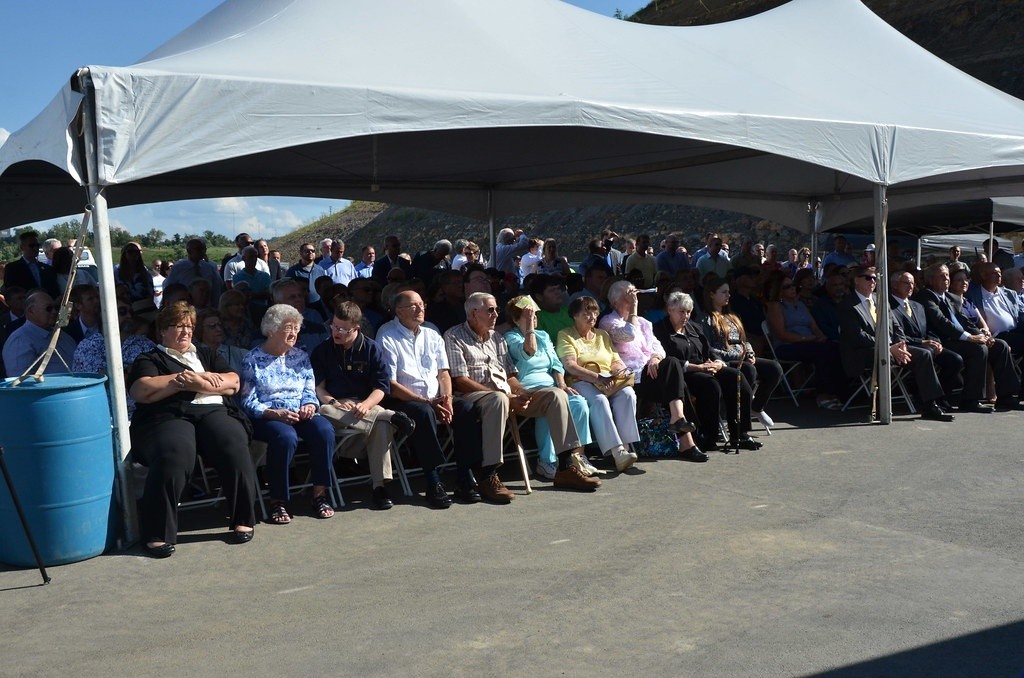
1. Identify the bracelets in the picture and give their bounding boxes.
[329,399,338,405]
[557,382,566,385]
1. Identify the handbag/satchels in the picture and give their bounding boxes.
[583,360,635,397]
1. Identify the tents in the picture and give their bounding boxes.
[811,197,1024,281]
[0,0,1024,555]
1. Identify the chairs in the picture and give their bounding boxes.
[178,319,1024,545]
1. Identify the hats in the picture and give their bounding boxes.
[130,298,159,321]
[863,243,875,252]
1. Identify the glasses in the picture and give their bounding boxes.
[857,274,876,281]
[302,249,315,253]
[329,322,356,334]
[202,321,224,328]
[488,307,499,313]
[166,322,196,333]
[33,305,58,312]
[23,243,41,248]
[464,250,478,256]
[399,303,427,311]
[781,283,797,290]
[239,241,255,246]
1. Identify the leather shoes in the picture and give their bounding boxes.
[667,412,774,463]
[142,542,176,558]
[921,392,1024,422]
[426,474,480,509]
[234,526,255,543]
[371,493,394,509]
[391,410,416,437]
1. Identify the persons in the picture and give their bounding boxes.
[690,232,1024,421]
[495,228,783,474]
[318,236,602,509]
[125,232,335,556]
[1,231,157,543]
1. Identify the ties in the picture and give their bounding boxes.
[866,296,964,330]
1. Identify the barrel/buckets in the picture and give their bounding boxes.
[0,372,114,568]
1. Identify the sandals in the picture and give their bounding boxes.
[270,496,334,524]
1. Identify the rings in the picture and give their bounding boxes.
[181,382,184,388]
[308,408,312,412]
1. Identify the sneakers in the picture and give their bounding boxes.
[478,474,516,504]
[536,452,637,491]
